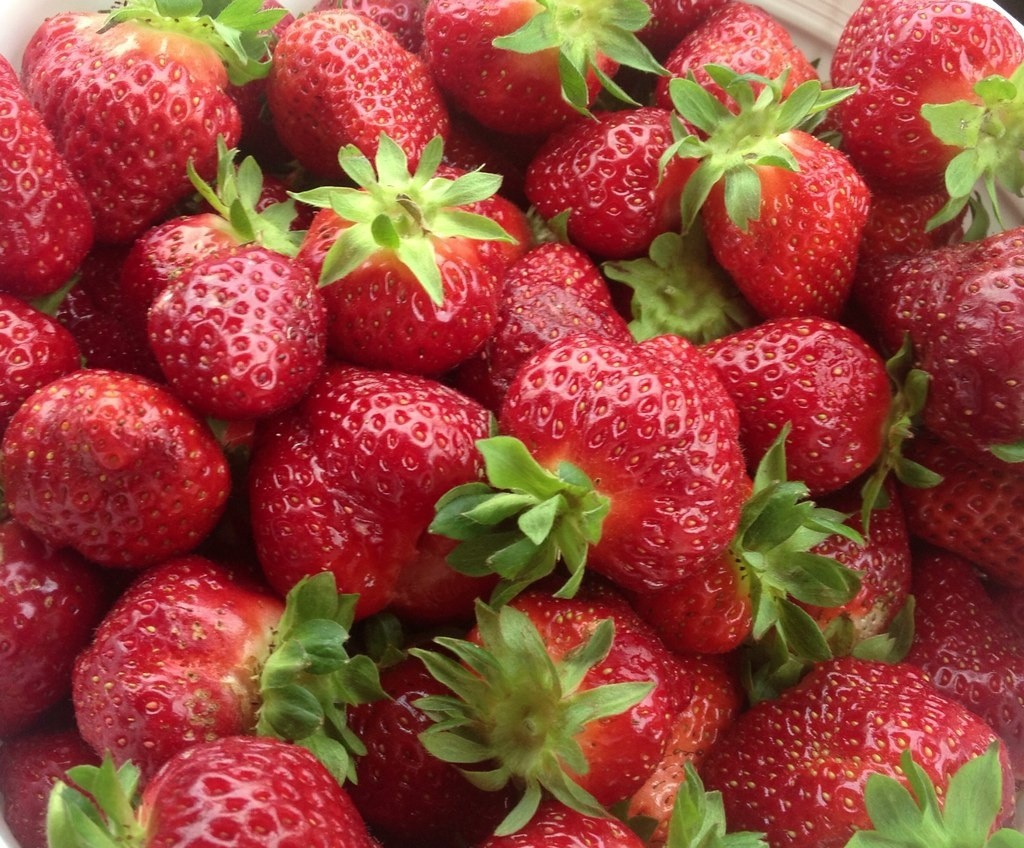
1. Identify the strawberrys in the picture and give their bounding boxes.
[0,0,1024,848]
